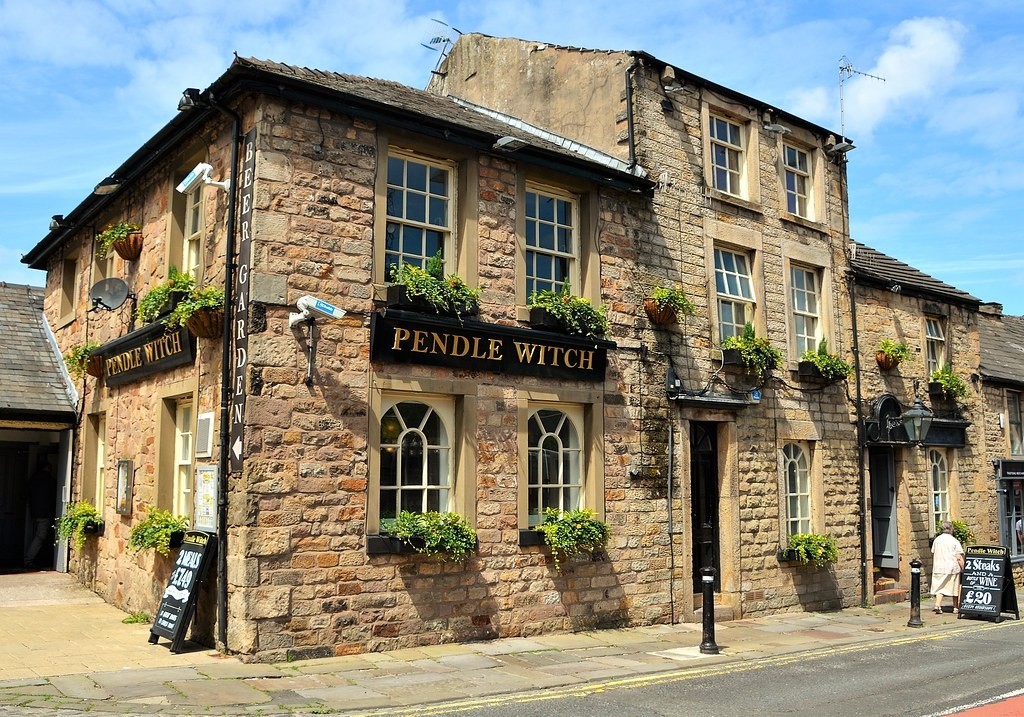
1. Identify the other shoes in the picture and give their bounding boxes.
[934,606,942,613]
[953,608,958,613]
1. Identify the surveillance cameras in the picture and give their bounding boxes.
[298,296,347,320]
[176,162,214,195]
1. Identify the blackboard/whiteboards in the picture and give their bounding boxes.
[959,545,1020,616]
[153,532,219,641]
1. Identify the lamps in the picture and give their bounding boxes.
[49,214,79,229]
[867,390,933,450]
[93,170,121,197]
[665,87,695,99]
[758,122,793,133]
[492,136,530,155]
[179,87,215,112]
[829,142,855,157]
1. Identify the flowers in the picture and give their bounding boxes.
[879,339,911,363]
[519,509,615,568]
[138,275,189,321]
[381,511,481,558]
[163,285,226,335]
[931,363,970,399]
[387,260,478,316]
[652,284,696,316]
[785,533,839,569]
[91,217,145,256]
[723,337,781,381]
[939,522,977,542]
[55,501,105,548]
[528,278,613,335]
[59,341,102,379]
[126,510,184,560]
[799,349,854,377]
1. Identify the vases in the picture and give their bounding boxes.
[77,355,103,379]
[724,348,777,370]
[929,382,958,400]
[386,286,476,315]
[876,351,904,368]
[800,366,847,384]
[929,537,963,550]
[367,530,472,553]
[188,304,224,343]
[518,527,608,546]
[155,533,184,550]
[82,519,103,538]
[781,548,833,568]
[645,301,682,325]
[530,307,608,333]
[113,230,144,260]
[149,288,193,321]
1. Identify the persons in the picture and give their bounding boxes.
[24,457,57,569]
[1016,518,1023,545]
[930,522,964,614]
